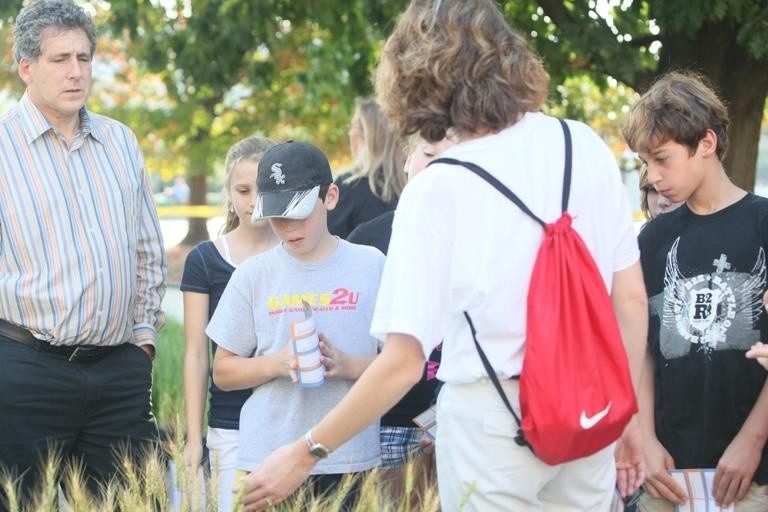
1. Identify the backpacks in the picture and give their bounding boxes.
[431,117,639,466]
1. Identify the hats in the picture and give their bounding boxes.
[250,140,332,224]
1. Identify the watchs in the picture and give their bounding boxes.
[300,428,330,461]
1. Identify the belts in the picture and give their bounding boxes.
[0,321,111,364]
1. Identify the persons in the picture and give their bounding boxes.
[742,340,768,374]
[639,160,689,229]
[176,132,285,512]
[616,67,768,512]
[320,98,418,240]
[341,120,467,508]
[2,0,171,511]
[233,1,653,512]
[199,139,389,511]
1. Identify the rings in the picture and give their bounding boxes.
[265,497,272,506]
